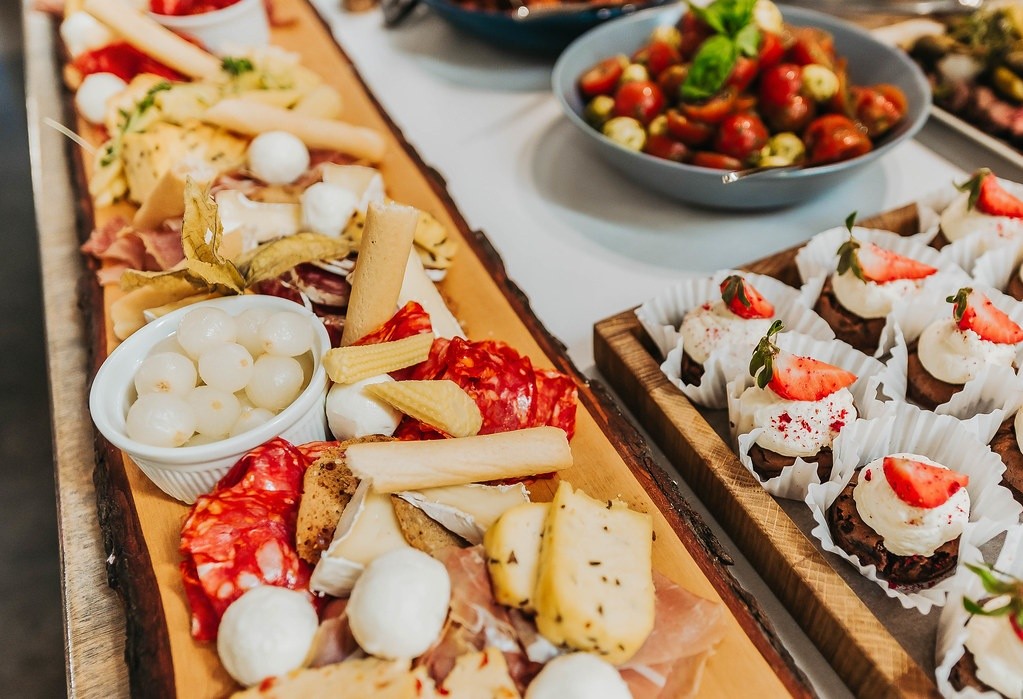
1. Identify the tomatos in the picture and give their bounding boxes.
[580,13,907,171]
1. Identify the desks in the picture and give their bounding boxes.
[19,0,1023,699]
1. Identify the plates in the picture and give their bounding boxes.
[871,0,1023,169]
[425,0,663,56]
[552,0,932,212]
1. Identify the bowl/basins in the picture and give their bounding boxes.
[146,0,270,60]
[87,293,332,507]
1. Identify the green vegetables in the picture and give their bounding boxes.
[679,0,764,99]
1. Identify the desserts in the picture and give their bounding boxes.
[632,166,1023,699]
